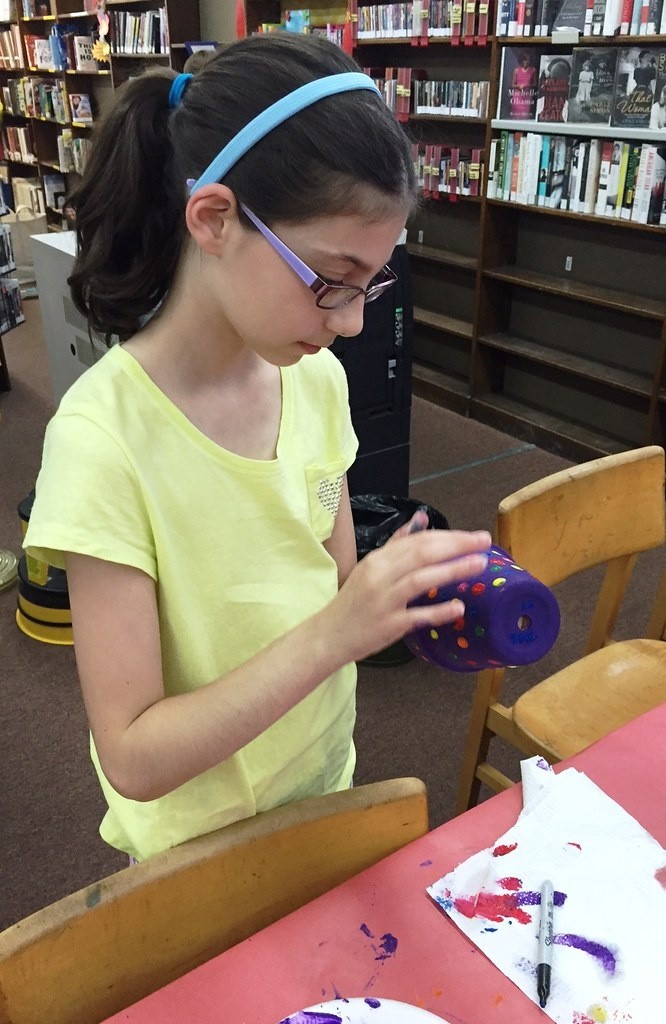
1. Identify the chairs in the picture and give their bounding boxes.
[0,776,427,1024]
[456,446,666,820]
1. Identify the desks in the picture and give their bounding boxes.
[100,705,666,1024]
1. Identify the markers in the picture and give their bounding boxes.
[537,880,553,1010]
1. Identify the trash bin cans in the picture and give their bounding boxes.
[349,494,449,668]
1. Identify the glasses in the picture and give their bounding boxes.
[187,177,398,310]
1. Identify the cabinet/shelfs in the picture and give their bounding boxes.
[0,0,200,234]
[242,0,666,460]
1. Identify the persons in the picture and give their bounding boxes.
[615,144,620,161]
[650,85,666,128]
[576,60,594,100]
[630,51,657,109]
[550,139,554,161]
[511,51,538,117]
[71,96,82,117]
[26,33,490,867]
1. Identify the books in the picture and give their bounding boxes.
[0,225,25,335]
[0,0,168,213]
[250,0,666,229]
